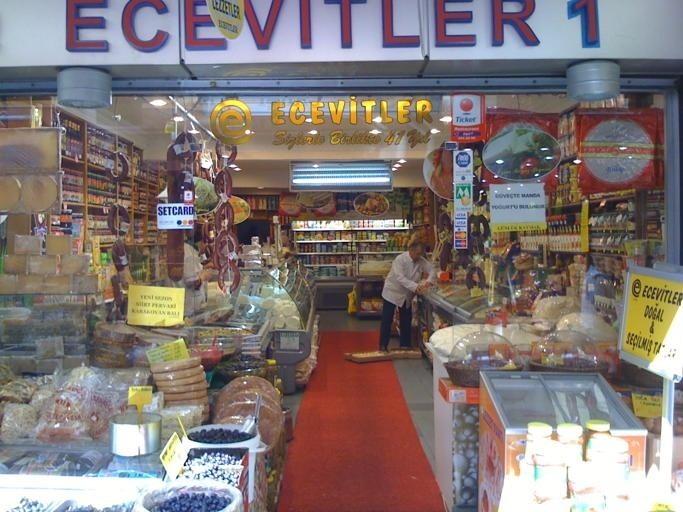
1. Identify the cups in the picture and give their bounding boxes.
[29,104,43,127]
[50,208,60,226]
[359,238,406,251]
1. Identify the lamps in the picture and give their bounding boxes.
[289,161,393,192]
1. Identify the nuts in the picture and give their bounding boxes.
[149,427,257,512]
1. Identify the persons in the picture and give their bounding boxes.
[377,239,438,355]
[181,230,216,313]
[429,139,455,201]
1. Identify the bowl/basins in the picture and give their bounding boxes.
[442,364,524,388]
[107,412,162,456]
[620,362,683,392]
[528,361,609,382]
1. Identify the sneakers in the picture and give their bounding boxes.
[399,343,414,350]
[380,344,388,354]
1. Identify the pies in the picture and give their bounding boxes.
[149,356,284,451]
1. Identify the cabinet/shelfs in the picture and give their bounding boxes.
[419,283,502,369]
[227,256,316,385]
[292,227,411,282]
[0,98,167,248]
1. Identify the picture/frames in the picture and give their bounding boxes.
[618,265,683,383]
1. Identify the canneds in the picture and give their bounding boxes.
[521,418,631,511]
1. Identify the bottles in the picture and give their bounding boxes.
[291,219,373,228]
[335,231,388,241]
[62,120,166,287]
[296,242,356,252]
